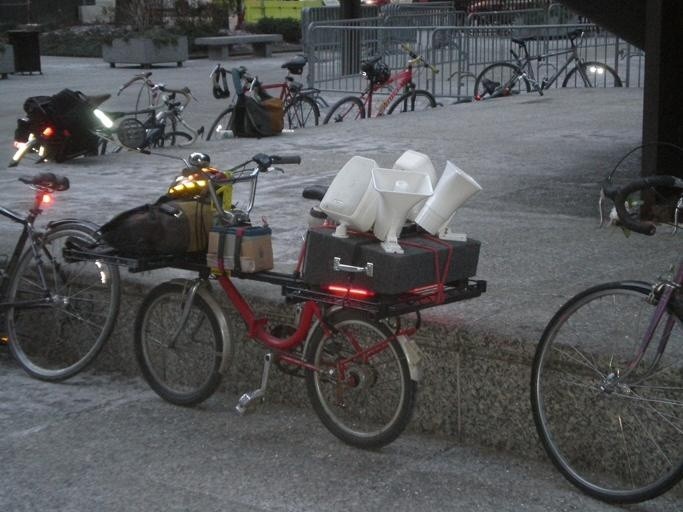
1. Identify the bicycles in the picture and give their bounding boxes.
[449,77,521,105]
[0,165,122,384]
[131,147,423,450]
[206,53,331,142]
[472,30,622,101]
[9,69,205,166]
[321,44,440,125]
[524,141,682,507]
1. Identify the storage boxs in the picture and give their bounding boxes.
[207,228,274,276]
